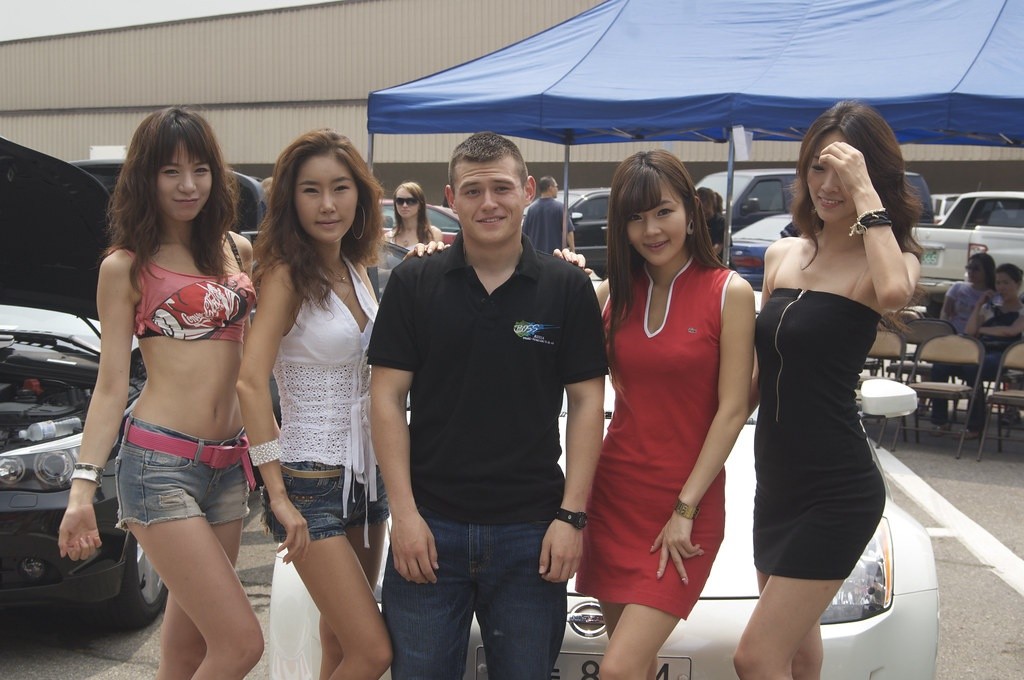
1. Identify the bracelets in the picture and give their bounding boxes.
[849,207,893,237]
[70,462,103,487]
[674,497,700,520]
[248,439,283,467]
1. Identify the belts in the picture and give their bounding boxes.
[124,416,257,491]
[280,464,341,478]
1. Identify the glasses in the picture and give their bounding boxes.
[395,198,419,205]
[965,266,981,272]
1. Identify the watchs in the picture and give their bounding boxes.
[554,507,589,530]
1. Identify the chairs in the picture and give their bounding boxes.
[859,305,1024,462]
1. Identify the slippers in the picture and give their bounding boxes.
[928,425,945,437]
[951,429,979,441]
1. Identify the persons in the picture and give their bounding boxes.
[236,126,454,680]
[379,181,444,249]
[521,175,576,254]
[369,131,608,680]
[552,147,755,680]
[57,107,265,680]
[697,186,733,266]
[927,253,1024,440]
[732,100,921,680]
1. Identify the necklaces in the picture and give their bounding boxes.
[403,238,409,246]
[322,263,349,284]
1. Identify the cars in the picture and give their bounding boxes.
[262,373,944,680]
[520,187,609,281]
[358,196,462,302]
[1,135,275,631]
[730,215,795,292]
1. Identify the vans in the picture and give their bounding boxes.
[696,165,935,242]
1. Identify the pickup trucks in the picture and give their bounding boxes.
[903,191,1024,286]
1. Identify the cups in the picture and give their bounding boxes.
[992,292,1004,307]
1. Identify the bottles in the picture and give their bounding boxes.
[18,415,83,442]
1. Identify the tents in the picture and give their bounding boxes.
[362,0,1024,308]
